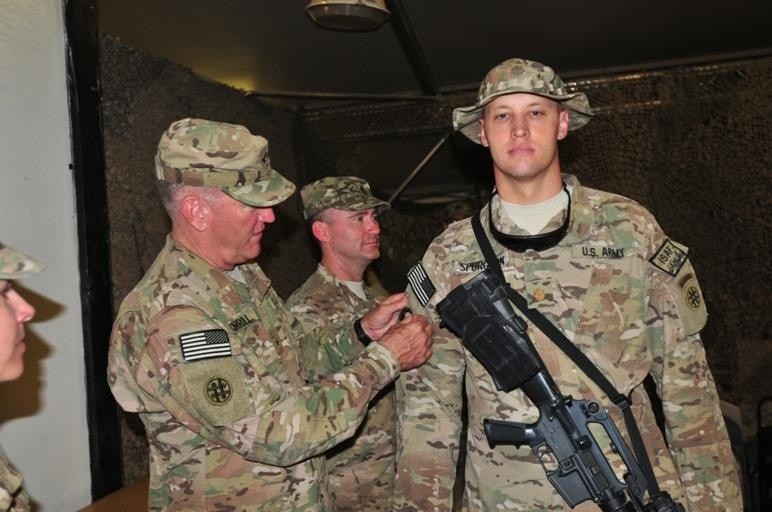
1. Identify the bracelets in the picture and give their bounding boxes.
[354,318,374,348]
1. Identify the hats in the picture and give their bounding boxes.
[154,117,297,209]
[453,59,593,144]
[1,241,46,280]
[300,175,391,222]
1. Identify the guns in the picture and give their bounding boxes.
[436,269,685,512]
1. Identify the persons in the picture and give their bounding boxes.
[107,115,434,511]
[0,241,49,512]
[283,174,401,510]
[399,56,743,511]
